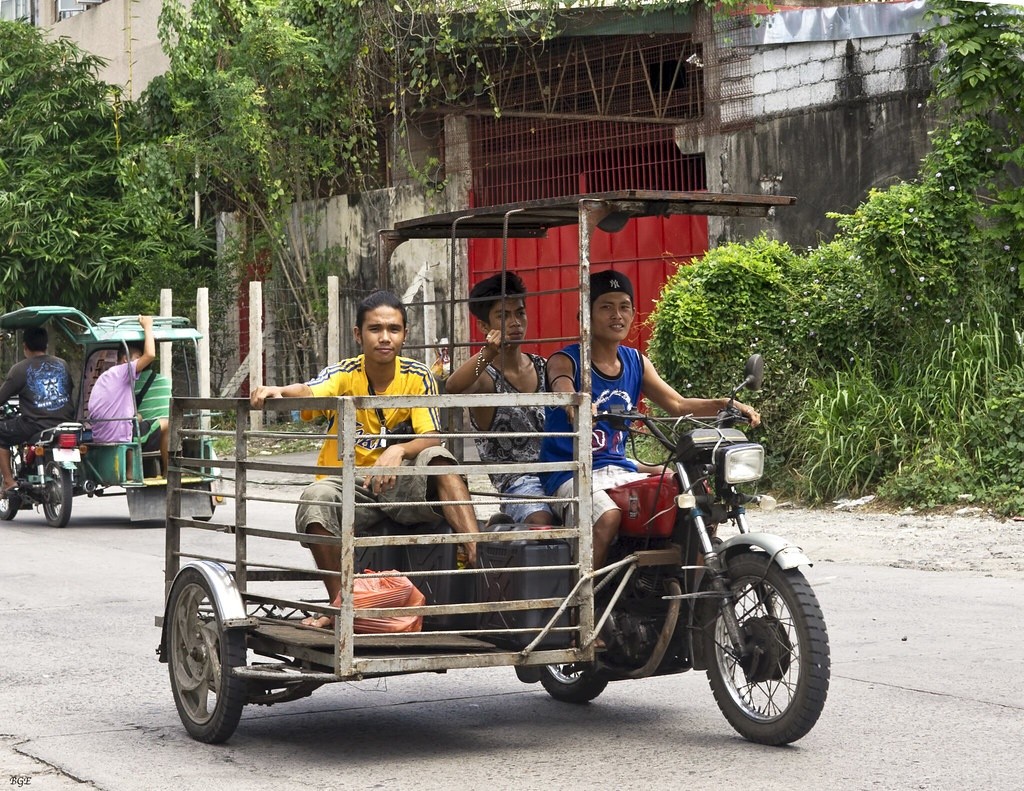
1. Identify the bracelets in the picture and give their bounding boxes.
[722,398,728,409]
[475,346,495,376]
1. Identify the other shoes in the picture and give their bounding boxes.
[0,482,20,492]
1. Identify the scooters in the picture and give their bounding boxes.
[0,305,227,528]
[156,186,829,747]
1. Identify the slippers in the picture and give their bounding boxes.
[300,612,334,630]
[484,513,515,529]
[571,626,608,653]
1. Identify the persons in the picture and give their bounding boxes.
[88,314,192,479]
[0,328,76,500]
[134,365,172,418]
[250,294,514,629]
[446,272,551,544]
[539,270,760,651]
[432,338,450,380]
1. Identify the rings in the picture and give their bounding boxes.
[746,410,751,414]
[755,413,759,416]
[487,335,492,338]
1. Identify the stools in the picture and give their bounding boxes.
[142,451,162,479]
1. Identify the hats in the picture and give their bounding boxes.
[576,269,633,322]
